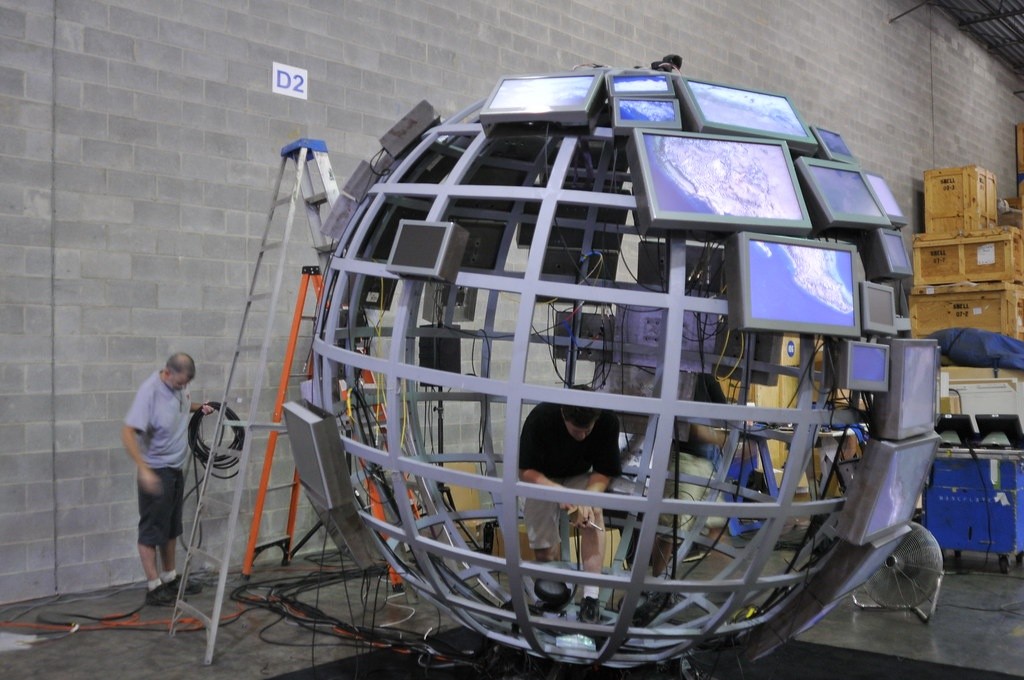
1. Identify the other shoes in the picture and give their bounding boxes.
[683,547,712,562]
[166,575,202,595]
[578,595,601,623]
[146,583,188,607]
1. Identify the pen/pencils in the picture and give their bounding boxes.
[588,520,604,532]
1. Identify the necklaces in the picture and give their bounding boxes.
[167,385,183,413]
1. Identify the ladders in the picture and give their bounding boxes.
[167,133,422,662]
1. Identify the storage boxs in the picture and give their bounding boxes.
[658,118,1024,556]
[441,462,628,583]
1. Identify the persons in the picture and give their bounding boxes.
[517,384,622,624]
[121,352,216,606]
[684,371,752,464]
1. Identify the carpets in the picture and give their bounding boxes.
[267,627,1024,680]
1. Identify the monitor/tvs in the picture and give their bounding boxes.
[280,68,939,660]
